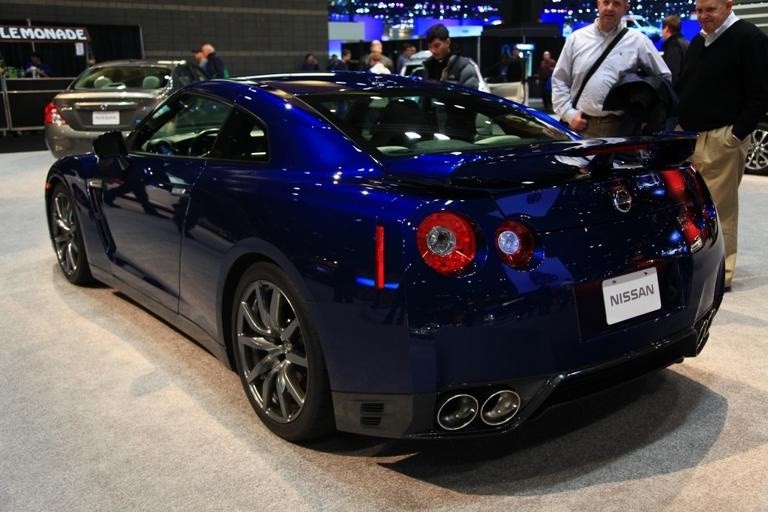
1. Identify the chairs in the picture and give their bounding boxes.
[94,75,112,88]
[340,97,434,147]
[142,75,161,91]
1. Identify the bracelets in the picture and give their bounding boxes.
[730,134,743,144]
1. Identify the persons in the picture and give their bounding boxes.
[202,42,226,78]
[677,0,768,292]
[24,52,52,77]
[659,14,690,132]
[192,48,208,71]
[548,0,674,140]
[421,23,479,143]
[305,39,556,113]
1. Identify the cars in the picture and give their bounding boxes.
[621,14,662,38]
[40,55,212,162]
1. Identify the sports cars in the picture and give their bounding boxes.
[43,67,728,453]
